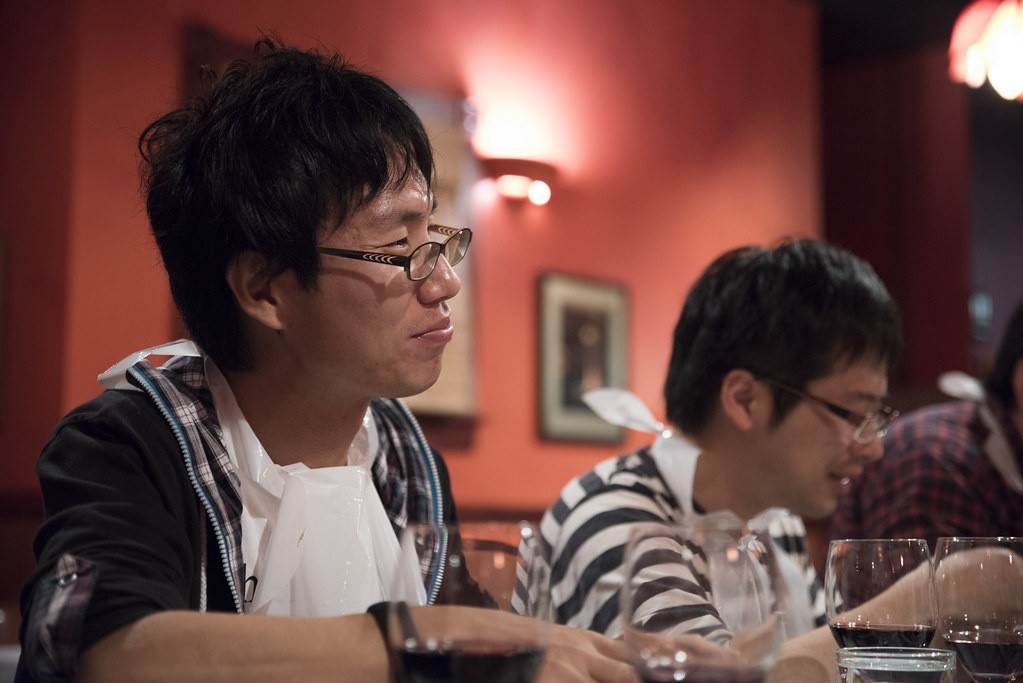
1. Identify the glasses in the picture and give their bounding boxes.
[754,374,900,443]
[303,223,473,281]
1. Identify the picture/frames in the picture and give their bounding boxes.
[392,88,482,425]
[538,269,630,442]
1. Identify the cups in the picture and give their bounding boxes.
[824,539,939,649]
[834,646,957,683]
[931,536,1023,683]
[385,522,552,682]
[621,525,782,683]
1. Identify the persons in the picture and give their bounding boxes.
[11,33,646,683]
[831,305,1023,610]
[512,235,1023,683]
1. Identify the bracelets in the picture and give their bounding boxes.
[365,600,422,658]
